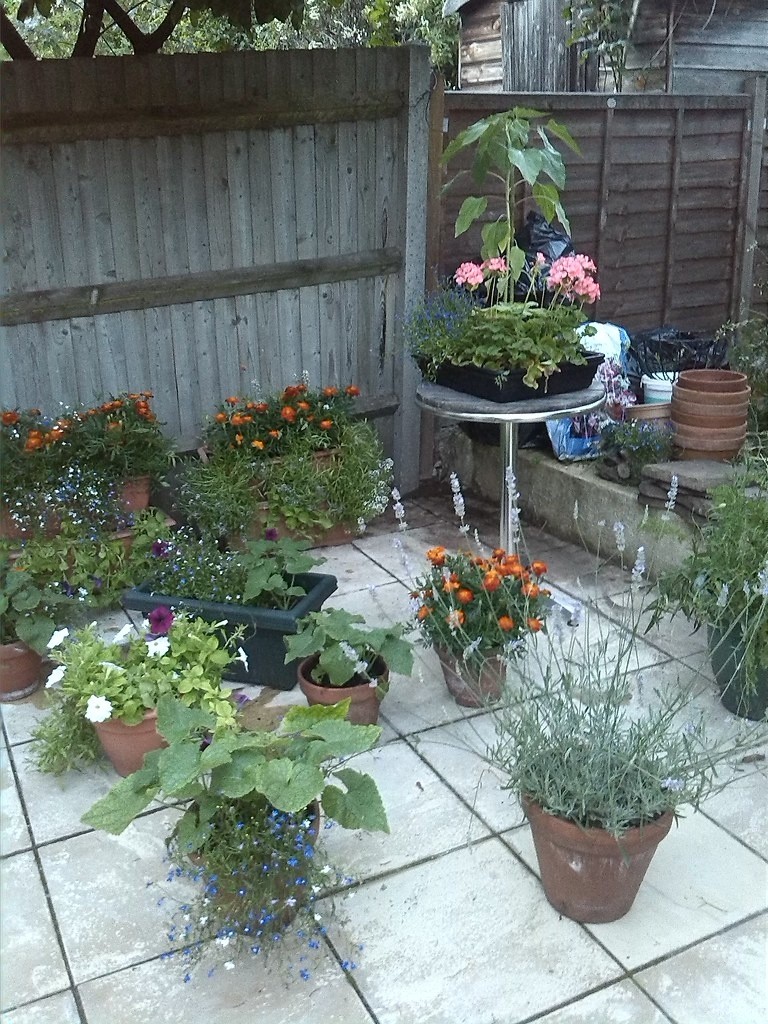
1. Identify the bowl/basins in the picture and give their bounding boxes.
[668,369,752,464]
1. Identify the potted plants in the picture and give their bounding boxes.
[283,608,418,727]
[645,461,768,723]
[0,572,86,702]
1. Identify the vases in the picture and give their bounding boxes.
[0,474,153,541]
[193,443,341,476]
[7,505,178,595]
[625,369,751,463]
[407,350,606,403]
[179,794,319,937]
[92,705,170,778]
[221,497,357,558]
[522,744,675,924]
[123,570,339,691]
[429,639,508,707]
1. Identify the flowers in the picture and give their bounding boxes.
[406,106,602,378]
[385,466,768,843]
[1,380,767,989]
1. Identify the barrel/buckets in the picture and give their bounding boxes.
[641,372,679,404]
[625,403,671,424]
